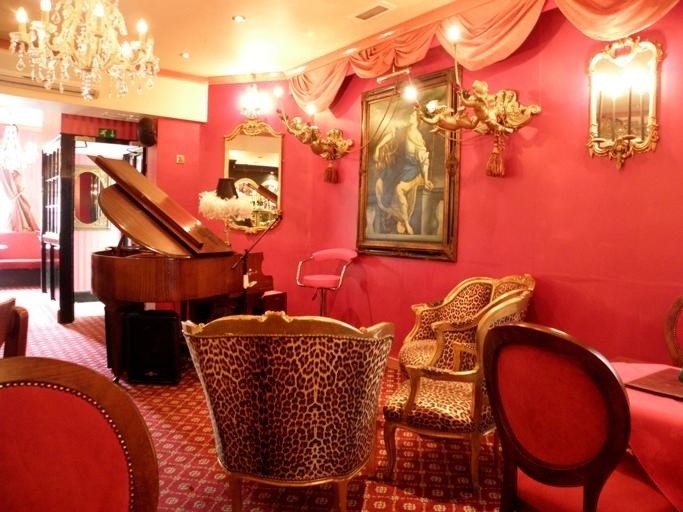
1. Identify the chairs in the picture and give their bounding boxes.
[661,300,679,366]
[181,310,396,511]
[382,291,530,490]
[481,320,672,509]
[400,273,535,389]
[0,355,159,512]
[0,297,28,358]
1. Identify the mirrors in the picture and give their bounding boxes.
[223,119,285,236]
[232,178,259,198]
[585,36,664,170]
[74,164,110,229]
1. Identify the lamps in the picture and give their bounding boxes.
[197,178,253,249]
[401,23,543,179]
[237,74,277,118]
[8,0,158,101]
[270,77,401,184]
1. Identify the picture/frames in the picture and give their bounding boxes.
[355,63,464,262]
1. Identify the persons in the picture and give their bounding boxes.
[373,108,435,235]
[0,123,40,232]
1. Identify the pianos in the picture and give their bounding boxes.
[86,155,272,385]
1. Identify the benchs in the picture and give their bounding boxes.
[44,248,61,270]
[0,230,41,271]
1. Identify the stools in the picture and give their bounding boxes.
[296,249,357,317]
